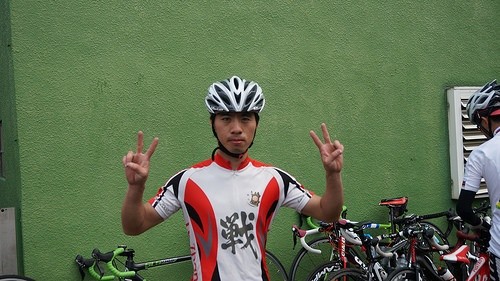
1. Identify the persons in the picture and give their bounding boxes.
[456,78,500,281]
[121,74,344,281]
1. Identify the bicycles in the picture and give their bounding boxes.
[0,197,497,281]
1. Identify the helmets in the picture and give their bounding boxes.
[204,75,267,114]
[466,78,500,123]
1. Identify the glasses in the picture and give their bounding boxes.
[476,111,491,131]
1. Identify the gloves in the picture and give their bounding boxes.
[472,219,492,237]
